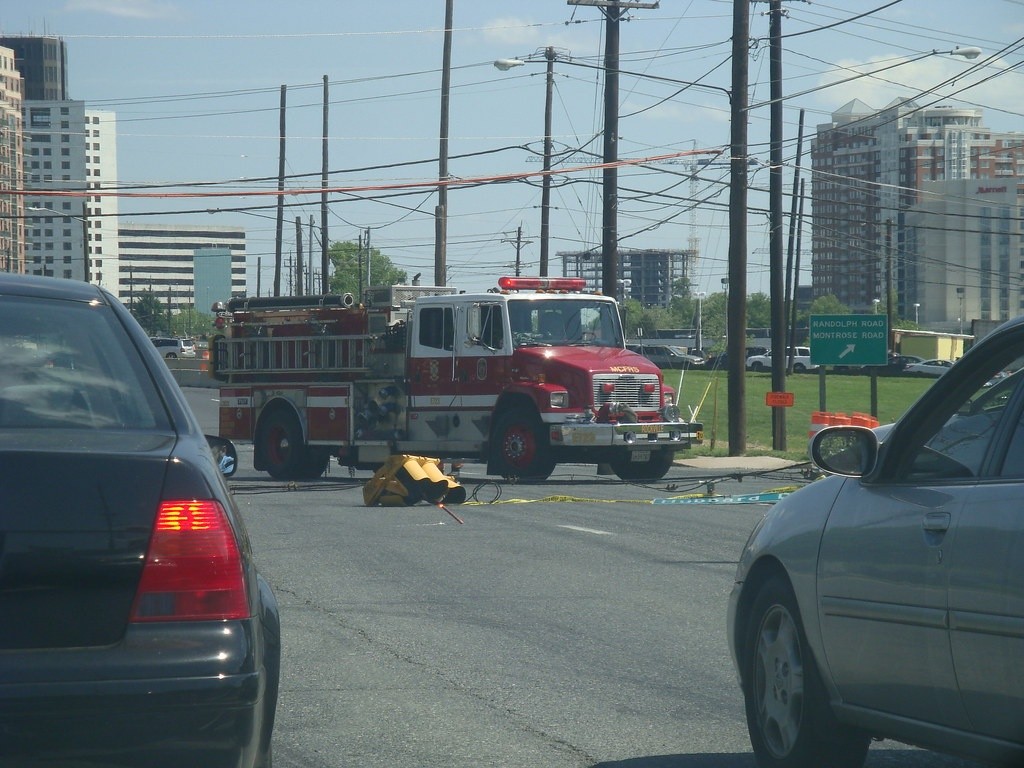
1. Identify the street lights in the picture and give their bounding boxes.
[913,303,920,331]
[491,46,982,455]
[873,298,880,314]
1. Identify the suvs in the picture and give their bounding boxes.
[746,347,820,373]
[633,344,704,369]
[149,337,196,359]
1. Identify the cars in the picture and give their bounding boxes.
[0,271,287,768]
[888,355,926,365]
[707,346,769,369]
[833,348,898,374]
[725,318,1024,768]
[903,359,954,374]
[868,365,1024,472]
[808,314,888,365]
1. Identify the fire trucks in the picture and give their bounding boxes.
[209,275,705,482]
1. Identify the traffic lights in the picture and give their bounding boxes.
[362,453,467,507]
